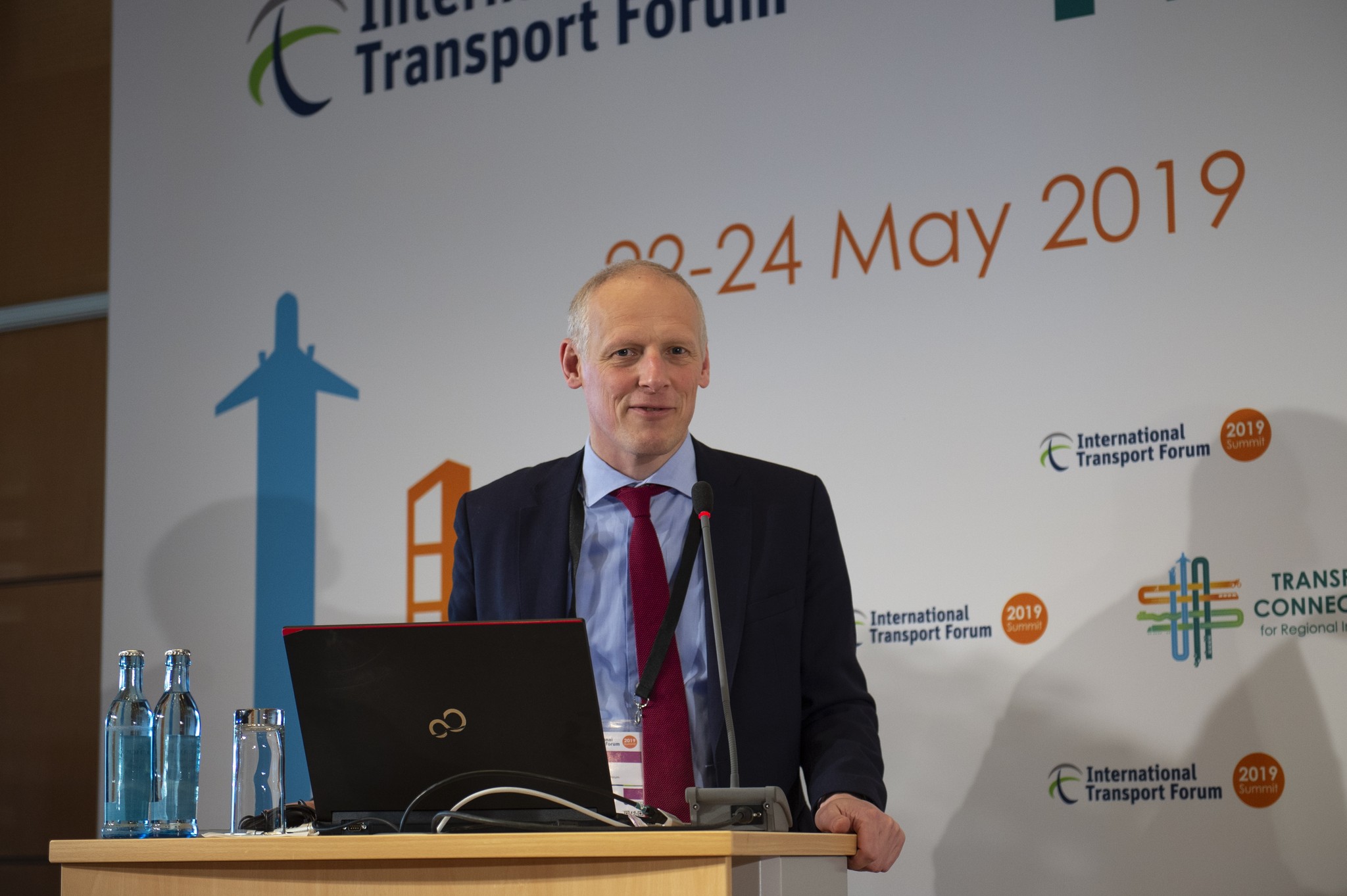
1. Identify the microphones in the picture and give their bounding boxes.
[672,481,796,833]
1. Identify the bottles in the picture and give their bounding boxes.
[152,649,202,838]
[101,650,153,839]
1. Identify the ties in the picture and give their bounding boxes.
[609,484,695,822]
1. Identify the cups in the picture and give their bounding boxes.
[230,709,286,836]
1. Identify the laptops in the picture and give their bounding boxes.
[281,618,620,830]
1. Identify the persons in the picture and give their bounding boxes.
[443,259,907,874]
